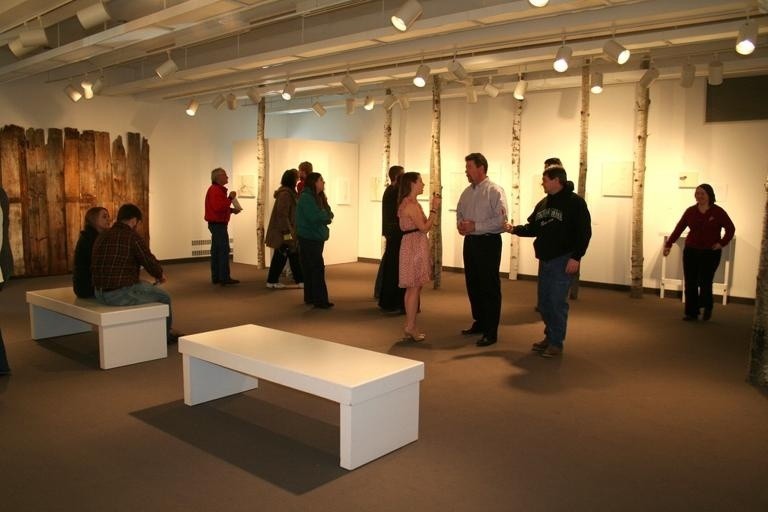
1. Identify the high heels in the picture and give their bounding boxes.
[404,326,425,342]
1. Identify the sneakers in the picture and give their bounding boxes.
[533,340,561,357]
[213,279,239,285]
[266,282,284,288]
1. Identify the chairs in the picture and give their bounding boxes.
[59,74,107,107]
[1,1,114,59]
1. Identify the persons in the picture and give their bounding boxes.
[662,183,735,322]
[0,186,14,376]
[527,158,575,311]
[73,206,110,298]
[374,166,421,315]
[504,167,592,358]
[92,204,185,345]
[456,153,508,346]
[205,167,241,285]
[396,173,440,341]
[265,161,334,308]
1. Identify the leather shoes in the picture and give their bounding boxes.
[476,335,496,346]
[463,327,483,335]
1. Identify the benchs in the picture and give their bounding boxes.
[176,322,427,473]
[21,283,172,372]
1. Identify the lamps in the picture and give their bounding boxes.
[155,50,178,82]
[180,36,729,117]
[388,2,427,33]
[734,2,761,57]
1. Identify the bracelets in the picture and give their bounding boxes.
[430,209,437,214]
[155,273,160,283]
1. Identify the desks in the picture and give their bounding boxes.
[655,229,738,308]
